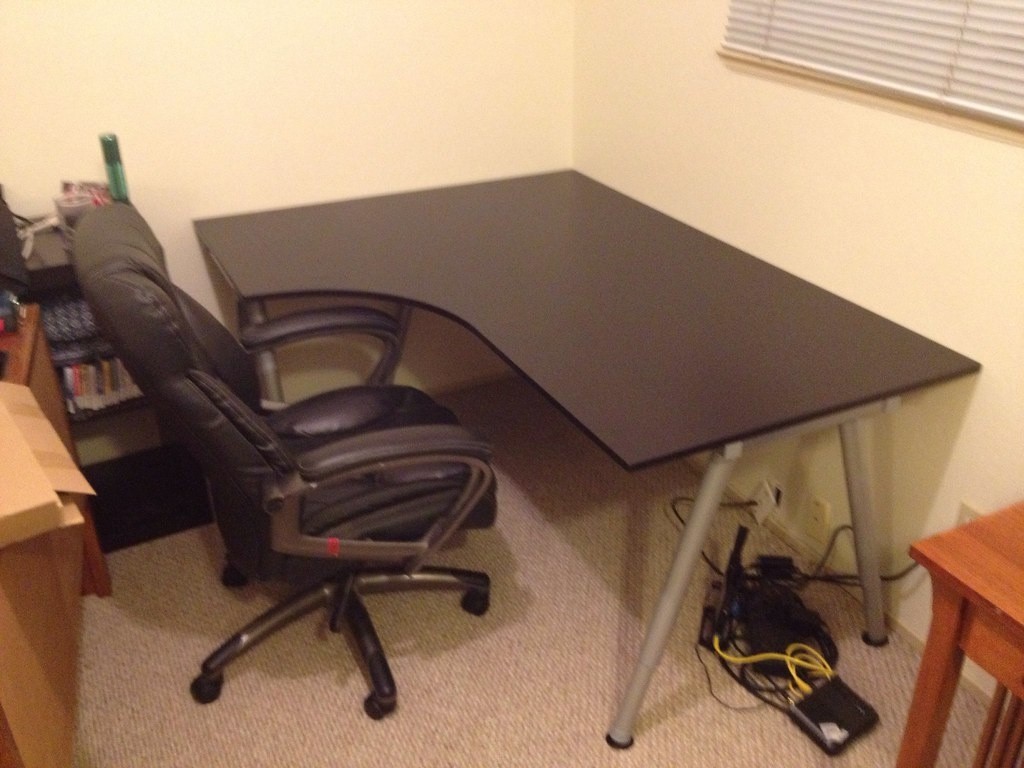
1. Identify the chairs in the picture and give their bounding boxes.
[71,199,499,721]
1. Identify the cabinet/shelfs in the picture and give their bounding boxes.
[16,214,214,552]
[0,303,116,768]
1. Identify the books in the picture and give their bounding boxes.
[63,356,143,414]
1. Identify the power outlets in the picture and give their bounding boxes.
[749,478,777,525]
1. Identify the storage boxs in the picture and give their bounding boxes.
[0,380,96,550]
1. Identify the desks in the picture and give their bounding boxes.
[896,498,1024,768]
[192,167,985,751]
